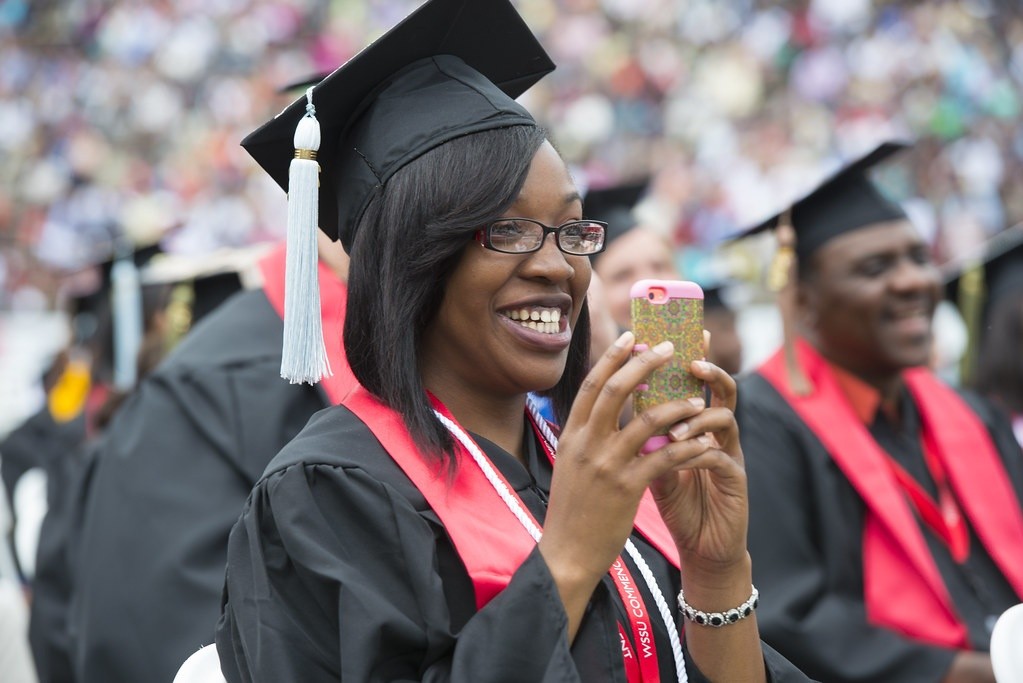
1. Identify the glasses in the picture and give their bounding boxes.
[471,217,609,254]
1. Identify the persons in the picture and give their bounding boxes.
[583,183,746,374]
[215,0,812,683]
[0,73,358,682]
[703,142,1023,683]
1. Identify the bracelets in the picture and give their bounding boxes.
[677,585,759,627]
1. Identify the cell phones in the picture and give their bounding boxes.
[626,277,706,452]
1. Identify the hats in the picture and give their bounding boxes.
[932,223,1023,390]
[720,138,913,396]
[577,175,652,265]
[240,0,557,387]
